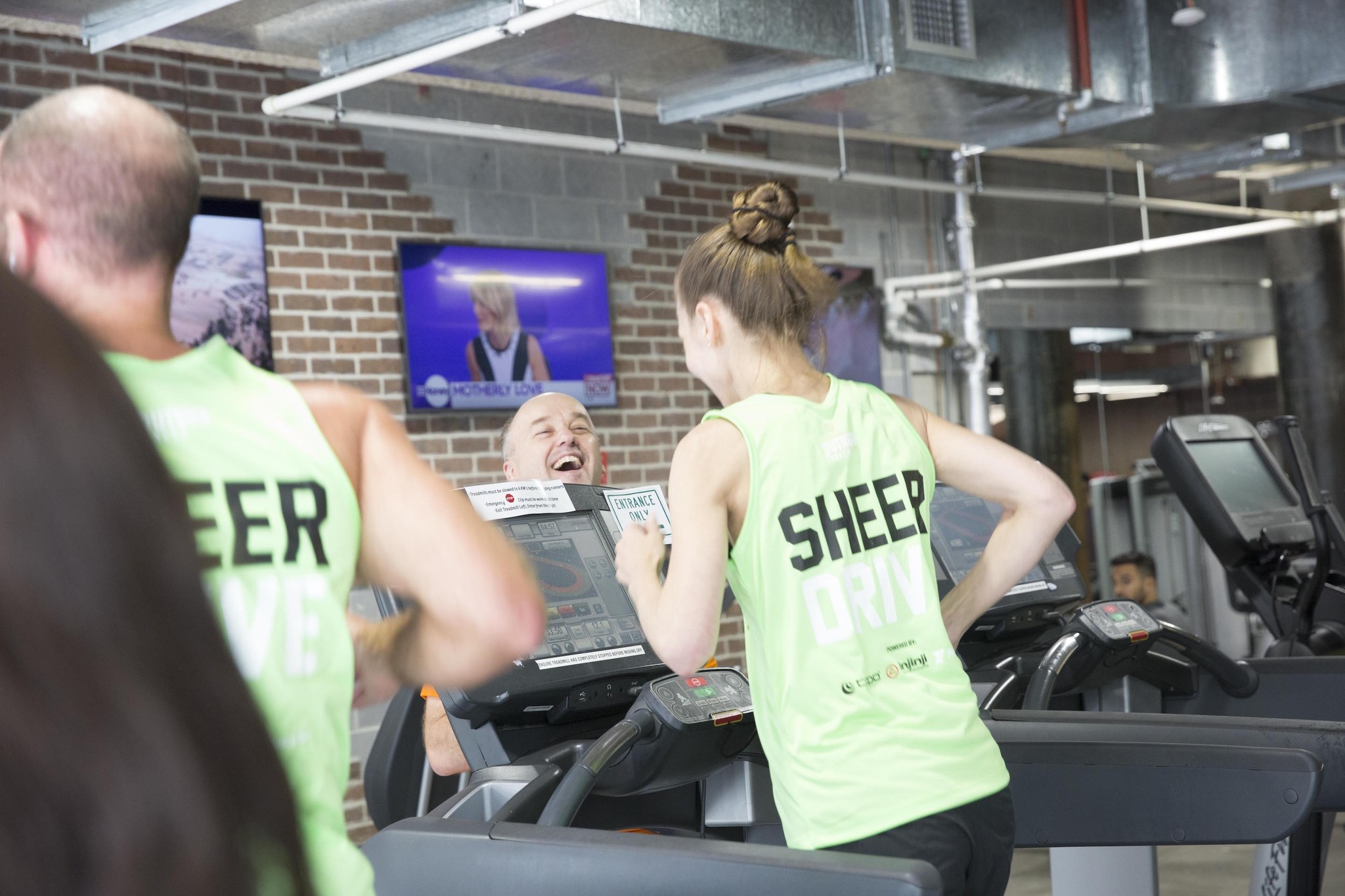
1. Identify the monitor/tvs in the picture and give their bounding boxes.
[809,261,883,390]
[367,481,682,730]
[1151,413,1316,569]
[396,237,621,416]
[927,479,1092,642]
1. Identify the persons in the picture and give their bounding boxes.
[462,267,550,382]
[615,184,1076,896]
[422,390,605,776]
[0,83,544,895]
[1111,552,1193,635]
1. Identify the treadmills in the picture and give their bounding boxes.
[354,413,1345,896]
